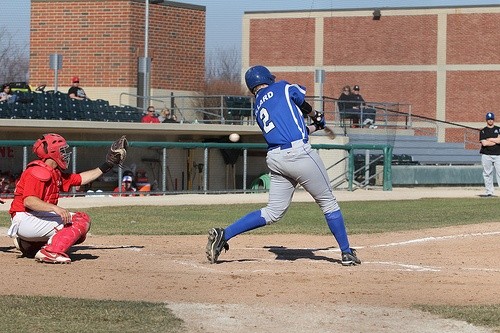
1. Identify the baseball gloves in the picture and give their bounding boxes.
[106,135,131,171]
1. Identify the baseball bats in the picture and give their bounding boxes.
[324,126,334,139]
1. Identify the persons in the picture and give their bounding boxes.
[141,106,160,123]
[57,182,92,197]
[0,83,15,102]
[337,86,369,127]
[113,168,162,196]
[0,170,23,204]
[479,113,500,197]
[68,77,86,100]
[158,107,177,123]
[205,67,362,266]
[351,85,377,129]
[10,133,128,264]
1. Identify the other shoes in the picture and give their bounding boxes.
[369,125,377,129]
[488,193,491,197]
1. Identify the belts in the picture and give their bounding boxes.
[281,139,308,150]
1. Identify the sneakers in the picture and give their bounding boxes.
[34,249,71,264]
[206,227,229,263]
[14,237,37,257]
[341,247,361,266]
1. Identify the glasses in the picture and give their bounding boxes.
[355,89,359,91]
[148,110,154,112]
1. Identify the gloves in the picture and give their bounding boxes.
[308,111,326,131]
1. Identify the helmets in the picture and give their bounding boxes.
[245,65,274,94]
[33,134,72,170]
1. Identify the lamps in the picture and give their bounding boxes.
[373,10,381,17]
[229,133,240,142]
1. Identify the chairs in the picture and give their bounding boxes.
[354,154,421,186]
[0,91,159,123]
[224,96,255,125]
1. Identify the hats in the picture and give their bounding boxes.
[73,77,79,82]
[485,112,494,118]
[343,86,352,91]
[123,176,133,182]
[354,85,359,89]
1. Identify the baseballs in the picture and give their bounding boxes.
[229,133,240,142]
